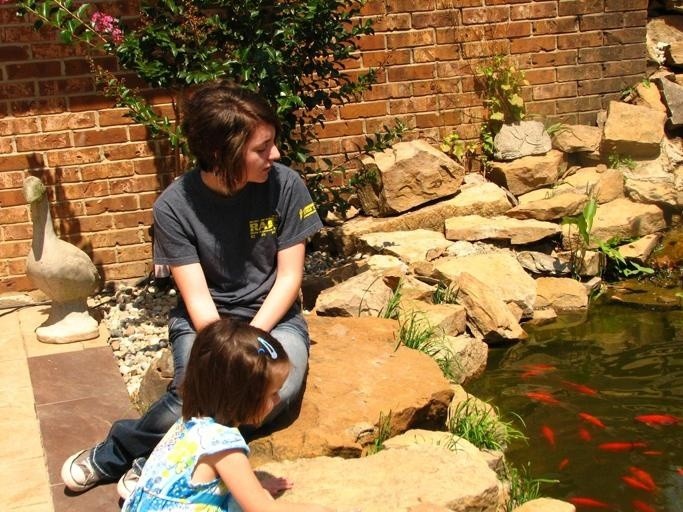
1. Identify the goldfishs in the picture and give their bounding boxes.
[518,363,601,406]
[542,412,683,512]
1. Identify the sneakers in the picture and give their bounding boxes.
[114,454,151,503]
[57,444,111,493]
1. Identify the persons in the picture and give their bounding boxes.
[121,319,362,512]
[60,77,323,500]
